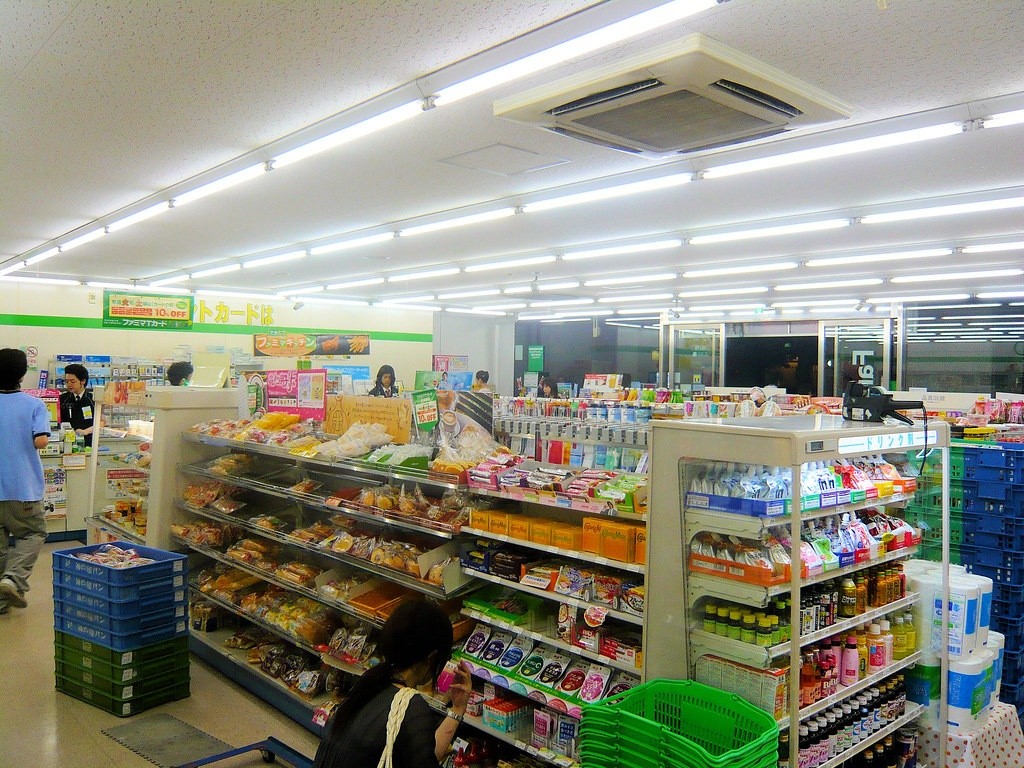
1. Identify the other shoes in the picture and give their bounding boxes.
[0,575,28,608]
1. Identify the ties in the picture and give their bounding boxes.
[76,396,80,401]
[385,390,390,396]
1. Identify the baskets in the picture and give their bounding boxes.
[577,677,778,768]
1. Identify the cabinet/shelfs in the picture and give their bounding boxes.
[485,380,970,473]
[173,422,490,766]
[646,420,949,766]
[88,383,239,552]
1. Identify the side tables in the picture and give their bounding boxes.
[443,461,649,767]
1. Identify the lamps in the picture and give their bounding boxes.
[0,0,1024,330]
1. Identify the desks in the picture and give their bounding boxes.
[911,701,1024,768]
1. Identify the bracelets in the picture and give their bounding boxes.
[448,711,464,722]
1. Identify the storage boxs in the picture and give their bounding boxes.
[902,438,1024,734]
[51,540,192,716]
[575,677,779,767]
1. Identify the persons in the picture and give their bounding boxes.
[313,599,472,768]
[166,361,193,387]
[439,372,449,389]
[58,364,96,447]
[369,365,398,398]
[476,370,492,392]
[542,377,561,399]
[0,348,51,614]
